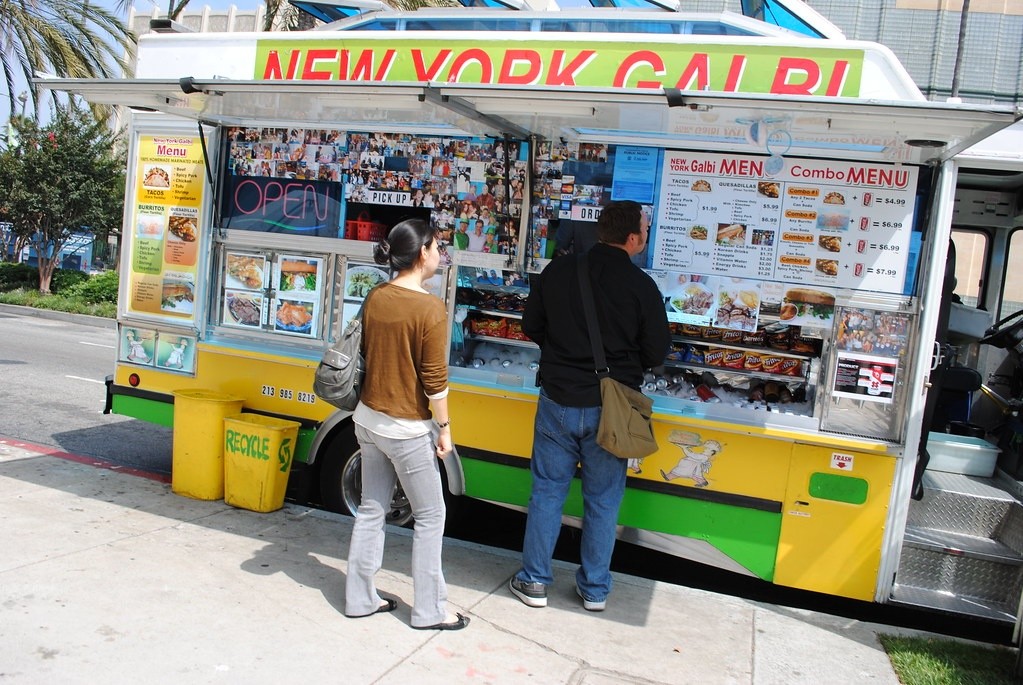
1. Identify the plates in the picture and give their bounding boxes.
[229,300,260,326]
[715,283,759,316]
[670,283,714,313]
[226,258,263,291]
[345,267,389,296]
[276,312,312,332]
[780,304,799,322]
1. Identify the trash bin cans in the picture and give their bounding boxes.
[224,412,303,512]
[171,386,246,501]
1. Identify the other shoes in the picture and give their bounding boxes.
[411,612,471,630]
[344,598,398,618]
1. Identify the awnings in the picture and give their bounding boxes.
[30,68,1023,305]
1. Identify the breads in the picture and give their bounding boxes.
[717,223,743,241]
[780,187,820,265]
[786,287,835,305]
[281,261,316,273]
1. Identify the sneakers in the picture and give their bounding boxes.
[573,567,606,610]
[508,571,548,607]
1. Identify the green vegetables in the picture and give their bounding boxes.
[280,273,316,291]
[784,297,834,320]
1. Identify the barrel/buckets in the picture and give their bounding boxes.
[950,421,986,440]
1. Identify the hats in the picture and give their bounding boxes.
[459,218,470,225]
[484,228,496,236]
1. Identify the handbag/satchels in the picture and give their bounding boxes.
[313,284,367,411]
[595,377,658,458]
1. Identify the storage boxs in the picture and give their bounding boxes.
[926,431,1003,477]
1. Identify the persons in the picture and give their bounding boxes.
[509,200,673,612]
[344,218,472,629]
[228,126,610,288]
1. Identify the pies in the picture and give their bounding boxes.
[692,180,711,192]
[143,167,170,187]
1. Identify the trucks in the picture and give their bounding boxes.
[27,0,1023,646]
[0,212,94,274]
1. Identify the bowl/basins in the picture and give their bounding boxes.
[925,431,1003,477]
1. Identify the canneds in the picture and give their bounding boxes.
[471,349,540,371]
[642,369,721,404]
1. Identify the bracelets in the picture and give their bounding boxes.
[437,418,450,428]
